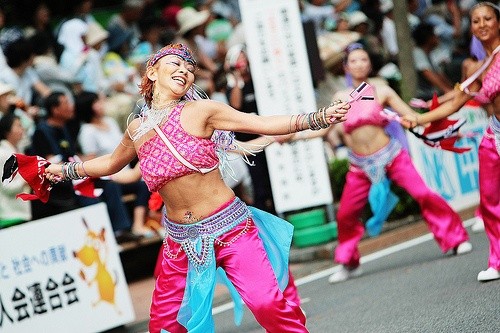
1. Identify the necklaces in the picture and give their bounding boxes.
[132,99,179,141]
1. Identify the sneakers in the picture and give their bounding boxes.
[478,265,500,281]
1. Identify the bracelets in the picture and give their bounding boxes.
[290,107,330,134]
[63,161,91,181]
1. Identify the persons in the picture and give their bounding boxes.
[59,19,125,94]
[407,0,500,99]
[86,0,274,224]
[43,43,351,333]
[249,42,473,282]
[73,91,166,236]
[35,90,144,242]
[401,4,500,281]
[74,131,299,305]
[300,0,399,87]
[0,0,92,227]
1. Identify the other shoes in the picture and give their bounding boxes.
[130,227,157,239]
[454,239,473,254]
[328,265,364,284]
[472,220,484,232]
